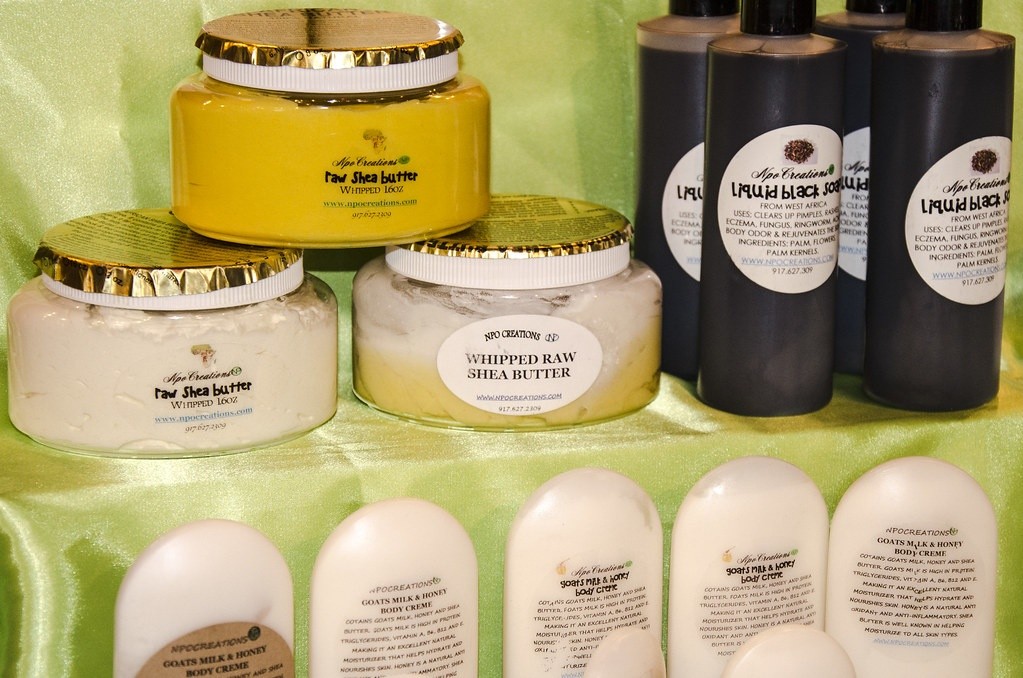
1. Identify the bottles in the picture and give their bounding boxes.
[169,7,490,248]
[9,209,340,461]
[629,1,1017,419]
[350,192,661,435]
